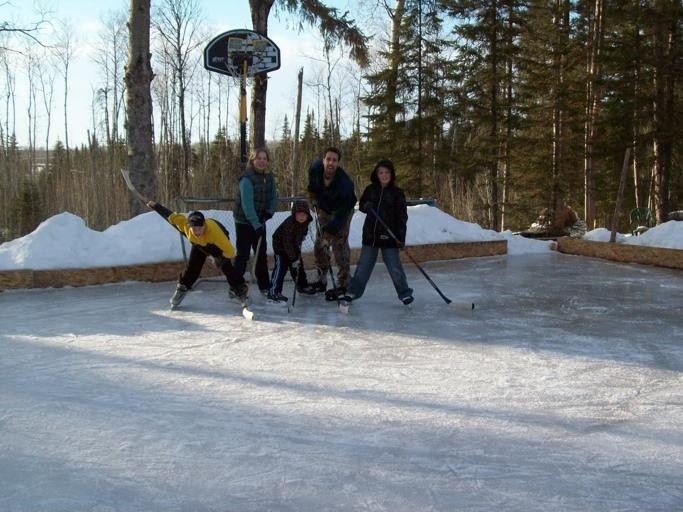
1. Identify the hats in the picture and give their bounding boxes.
[188,210,205,227]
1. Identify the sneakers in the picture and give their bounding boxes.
[402,295,414,304]
[297,279,357,301]
[229,285,288,301]
[170,284,188,305]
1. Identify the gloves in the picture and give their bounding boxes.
[306,192,319,212]
[259,211,272,223]
[315,235,330,249]
[217,254,230,265]
[256,226,265,239]
[291,260,302,270]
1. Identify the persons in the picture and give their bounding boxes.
[307,147,357,301]
[228,146,276,300]
[147,202,252,308]
[268,199,315,302]
[340,160,414,304]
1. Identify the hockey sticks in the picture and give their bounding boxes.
[242,216,263,320]
[370,208,475,311]
[287,246,302,311]
[121,170,187,238]
[313,206,351,314]
